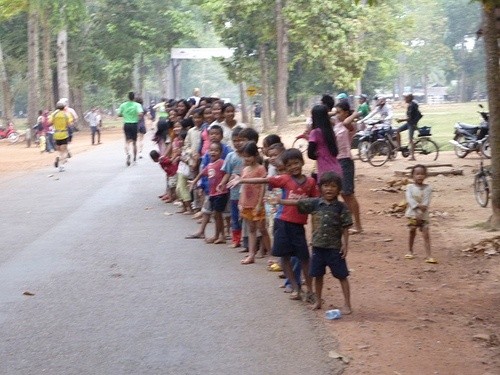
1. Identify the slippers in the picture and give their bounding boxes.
[268,262,284,270]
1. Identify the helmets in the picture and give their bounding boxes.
[55,100,66,108]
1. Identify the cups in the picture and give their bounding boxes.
[324,309,341,320]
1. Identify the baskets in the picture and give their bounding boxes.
[418,126,431,136]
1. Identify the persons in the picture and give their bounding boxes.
[116,88,437,316]
[34,98,102,171]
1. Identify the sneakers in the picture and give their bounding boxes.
[54,156,60,168]
[59,165,66,172]
[126,154,131,166]
